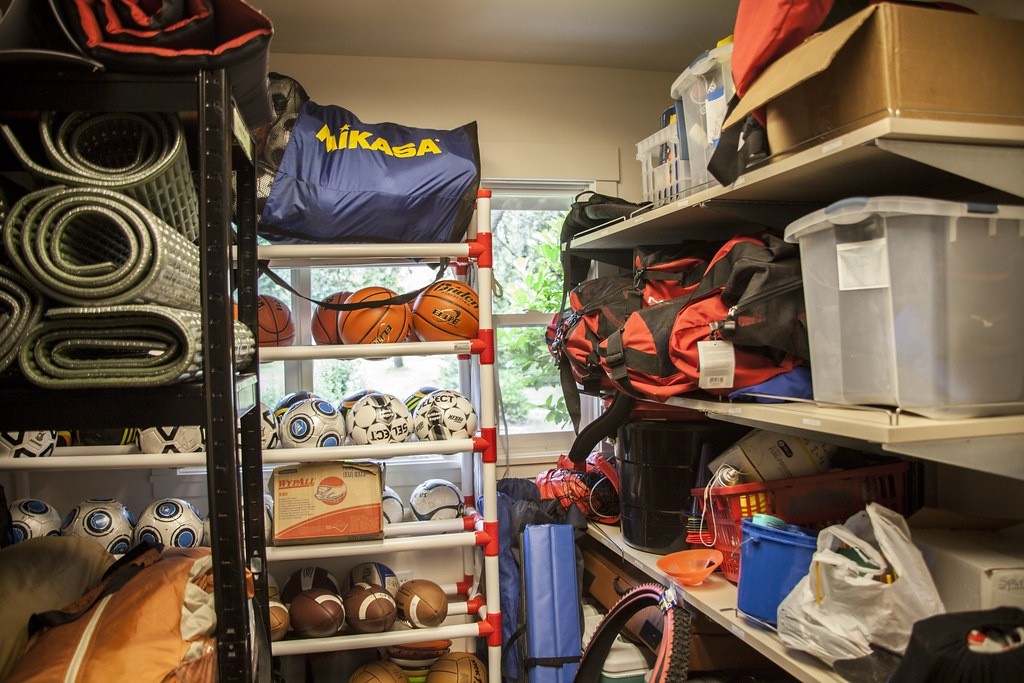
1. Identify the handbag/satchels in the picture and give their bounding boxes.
[560,231,812,464]
[496,454,625,683]
[888,606,1024,683]
[260,96,480,310]
[707,1,979,188]
[5,540,256,683]
[776,502,946,668]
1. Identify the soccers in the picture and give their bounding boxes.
[0,386,478,556]
[267,562,400,604]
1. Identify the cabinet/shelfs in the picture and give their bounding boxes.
[564,116,1024,683]
[0,66,272,683]
[0,190,502,683]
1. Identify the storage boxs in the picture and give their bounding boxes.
[904,506,1024,613]
[719,4,1024,162]
[707,426,842,484]
[672,42,735,193]
[692,462,931,581]
[578,594,655,683]
[782,195,1024,423]
[636,124,677,209]
[268,462,388,546]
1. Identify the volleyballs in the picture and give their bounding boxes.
[349,652,489,683]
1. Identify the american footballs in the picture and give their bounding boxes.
[267,579,447,641]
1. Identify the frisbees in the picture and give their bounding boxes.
[387,640,452,682]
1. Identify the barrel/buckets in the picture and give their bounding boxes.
[604,399,746,555]
[734,517,818,623]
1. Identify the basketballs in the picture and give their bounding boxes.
[310,280,480,363]
[232,295,296,364]
